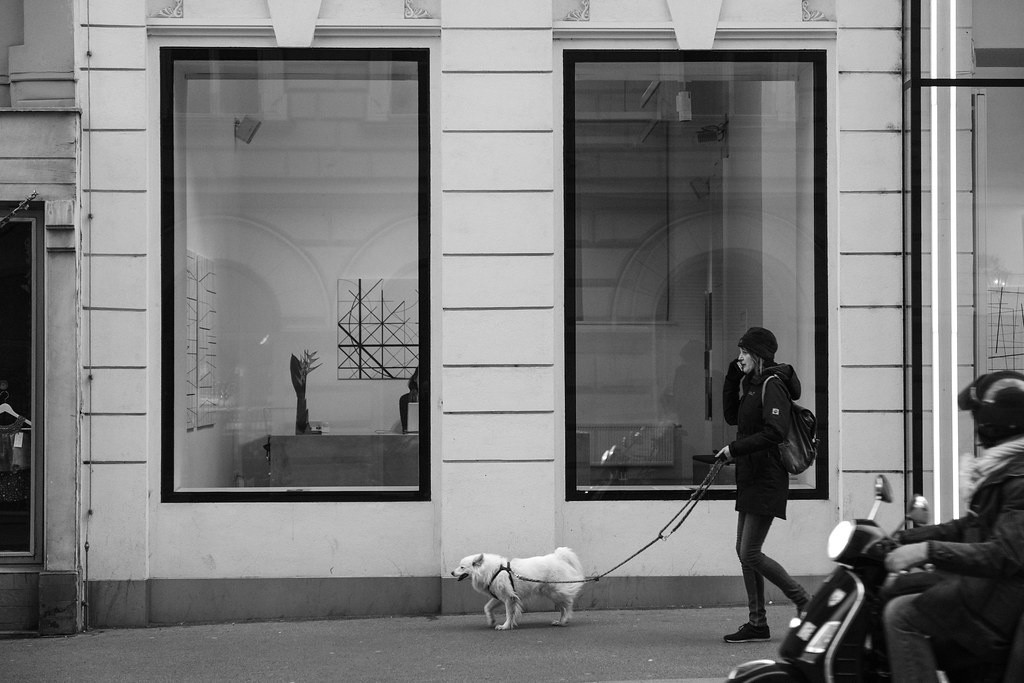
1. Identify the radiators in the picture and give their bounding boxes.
[580,422,684,466]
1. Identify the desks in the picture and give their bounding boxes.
[269,430,590,487]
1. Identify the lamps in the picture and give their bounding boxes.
[233,115,263,145]
[695,124,729,144]
[690,175,711,201]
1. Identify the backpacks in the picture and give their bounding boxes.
[762,373,820,474]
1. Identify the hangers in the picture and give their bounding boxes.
[0,391,32,431]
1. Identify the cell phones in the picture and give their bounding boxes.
[736,360,744,372]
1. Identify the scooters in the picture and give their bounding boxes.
[724,476,1022,683]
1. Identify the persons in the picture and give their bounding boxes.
[883,369,1024,683]
[714,327,814,643]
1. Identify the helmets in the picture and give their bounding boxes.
[957,371,1024,449]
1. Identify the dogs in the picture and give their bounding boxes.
[451,546,586,631]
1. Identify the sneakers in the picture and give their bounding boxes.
[723,622,772,642]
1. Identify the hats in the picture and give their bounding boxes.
[739,328,779,362]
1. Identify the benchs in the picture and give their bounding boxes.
[693,454,735,486]
